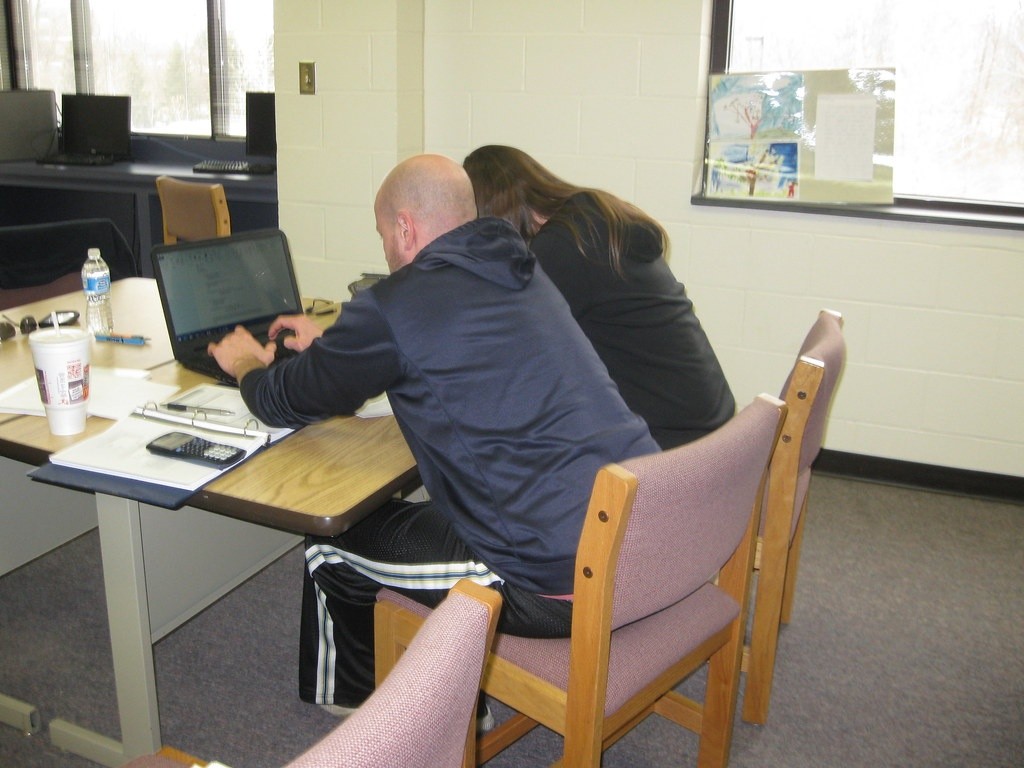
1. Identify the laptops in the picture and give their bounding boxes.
[151,231,302,387]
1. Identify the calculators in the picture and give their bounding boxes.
[146,432,247,470]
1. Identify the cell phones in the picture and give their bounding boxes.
[39,311,79,327]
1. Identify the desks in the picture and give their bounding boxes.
[0,156,278,279]
[0,279,417,768]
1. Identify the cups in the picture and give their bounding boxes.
[28,326,92,437]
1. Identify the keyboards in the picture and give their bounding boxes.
[43,154,114,165]
[193,160,276,174]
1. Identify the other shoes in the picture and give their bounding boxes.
[321,702,359,717]
[475,705,493,733]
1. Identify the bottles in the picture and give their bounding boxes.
[81,248,115,336]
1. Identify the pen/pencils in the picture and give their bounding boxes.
[160,403,235,416]
[95,334,144,346]
[111,332,144,340]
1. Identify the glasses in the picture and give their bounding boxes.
[0,315,37,341]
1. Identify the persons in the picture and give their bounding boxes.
[209,153,662,733]
[463,145,735,450]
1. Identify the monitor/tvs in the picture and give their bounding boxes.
[62,94,130,158]
[246,92,277,157]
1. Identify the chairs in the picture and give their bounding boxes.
[0,219,138,315]
[374,392,790,767]
[700,309,847,725]
[155,175,231,245]
[116,577,502,768]
